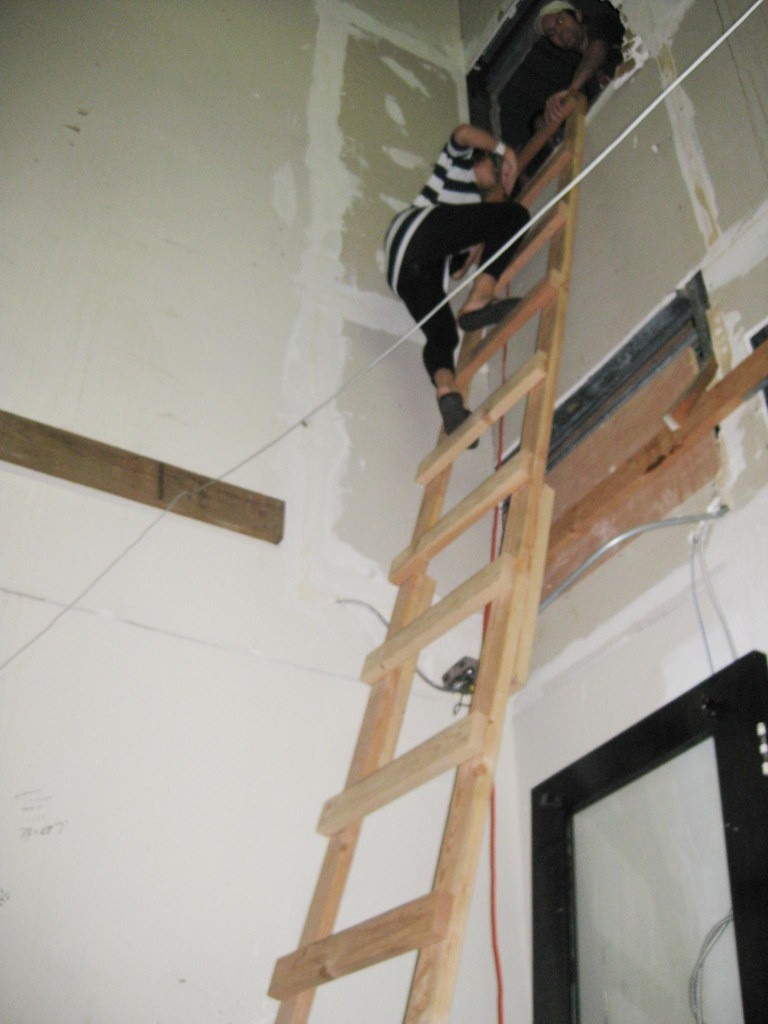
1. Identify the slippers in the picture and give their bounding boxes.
[458,296,524,332]
[444,409,479,449]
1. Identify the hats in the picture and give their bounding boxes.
[533,0,575,36]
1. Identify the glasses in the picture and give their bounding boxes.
[546,14,573,39]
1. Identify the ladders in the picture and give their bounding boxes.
[266,96,583,1024]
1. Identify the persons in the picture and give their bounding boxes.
[380,121,519,452]
[533,0,624,150]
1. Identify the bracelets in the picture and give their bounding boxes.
[493,142,506,157]
